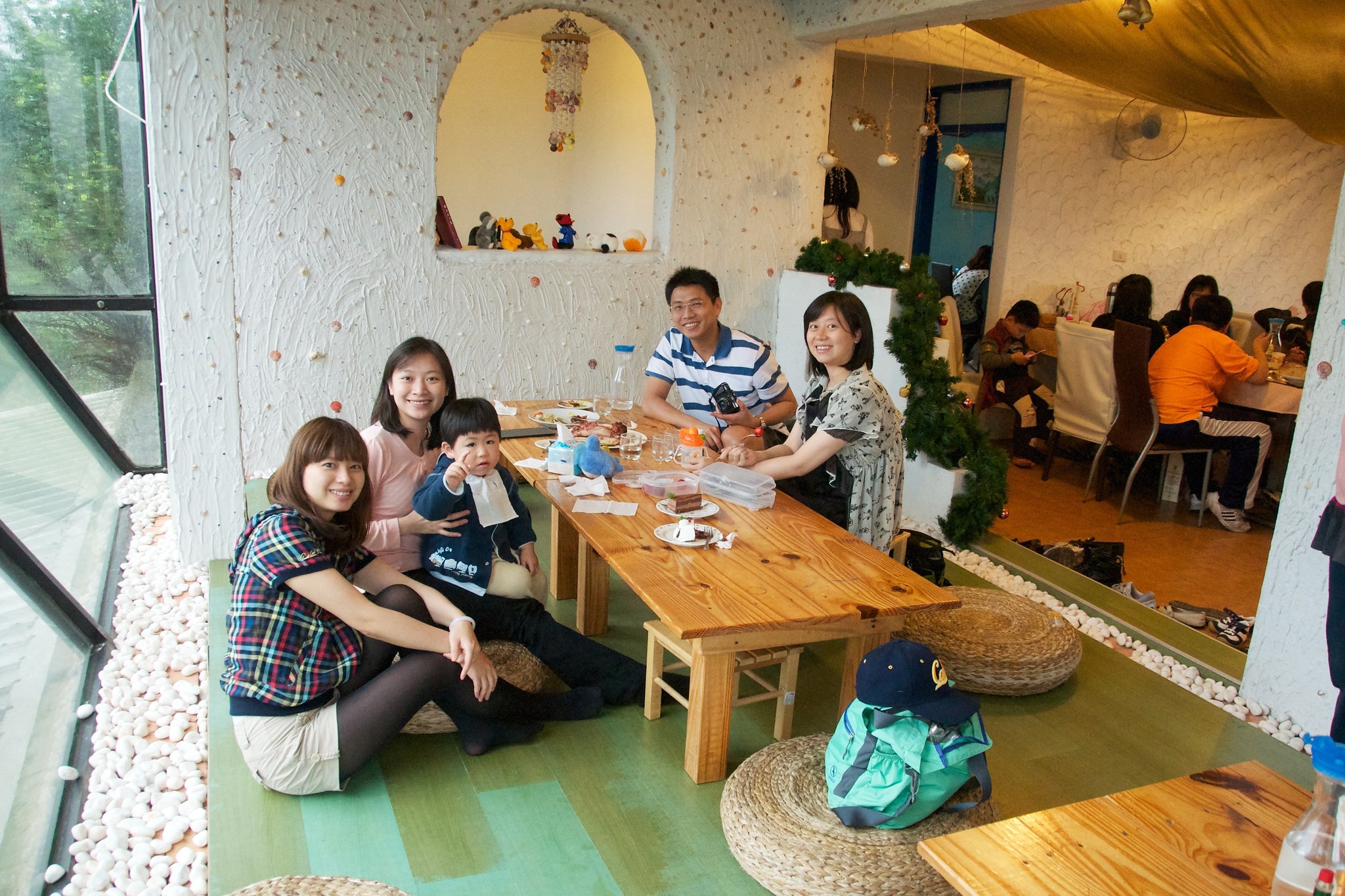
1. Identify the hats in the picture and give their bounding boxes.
[855,638,983,727]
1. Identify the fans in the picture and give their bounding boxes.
[1111,96,1187,161]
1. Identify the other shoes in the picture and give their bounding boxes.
[1157,604,1206,628]
[1168,599,1232,623]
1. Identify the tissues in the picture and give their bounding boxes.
[546,420,590,479]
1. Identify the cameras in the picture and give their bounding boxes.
[711,382,740,415]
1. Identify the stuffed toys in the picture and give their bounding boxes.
[471,210,619,252]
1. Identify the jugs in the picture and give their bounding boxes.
[610,345,635,410]
[1270,732,1345,896]
[1267,318,1285,352]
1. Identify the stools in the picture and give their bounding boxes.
[642,618,805,743]
[887,527,910,566]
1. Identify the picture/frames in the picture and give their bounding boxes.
[951,147,1003,212]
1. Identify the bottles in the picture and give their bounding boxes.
[697,429,706,456]
[1313,869,1334,896]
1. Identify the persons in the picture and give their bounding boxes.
[952,244,992,364]
[1092,273,1165,365]
[358,337,690,704]
[413,397,547,608]
[220,417,602,796]
[1255,280,1324,346]
[718,290,903,559]
[978,301,1055,469]
[1279,328,1310,358]
[1161,274,1233,339]
[1289,347,1308,368]
[642,262,799,451]
[1146,292,1270,534]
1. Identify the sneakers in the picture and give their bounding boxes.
[1208,614,1256,636]
[1206,491,1252,532]
[1216,620,1249,647]
[1043,542,1084,568]
[1190,494,1207,510]
[1111,581,1157,611]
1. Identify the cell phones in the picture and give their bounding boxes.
[500,426,554,441]
[1027,349,1047,358]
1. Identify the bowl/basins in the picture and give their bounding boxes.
[699,462,777,511]
[641,470,699,499]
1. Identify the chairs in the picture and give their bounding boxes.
[1229,317,1253,350]
[1043,318,1163,503]
[931,262,985,373]
[936,295,1024,461]
[1230,310,1282,422]
[1080,318,1214,529]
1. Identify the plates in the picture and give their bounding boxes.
[654,523,721,547]
[528,409,600,427]
[1278,373,1304,384]
[656,499,720,519]
[557,400,593,409]
[535,439,557,448]
[569,424,648,448]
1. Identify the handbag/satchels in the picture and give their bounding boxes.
[824,679,993,830]
[1070,537,1125,588]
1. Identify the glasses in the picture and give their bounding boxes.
[668,295,715,313]
[1191,292,1202,299]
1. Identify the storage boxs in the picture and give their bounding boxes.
[698,462,777,512]
[643,468,700,498]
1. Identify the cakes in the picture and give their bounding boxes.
[666,492,702,515]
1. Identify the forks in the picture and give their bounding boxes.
[703,526,713,550]
[542,441,555,454]
[662,502,710,508]
[716,434,760,460]
[557,419,580,425]
[569,416,611,422]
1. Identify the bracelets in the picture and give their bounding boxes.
[449,616,476,632]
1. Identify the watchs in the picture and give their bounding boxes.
[755,414,767,430]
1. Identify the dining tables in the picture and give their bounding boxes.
[917,761,1344,895]
[475,396,961,784]
[1025,312,1309,415]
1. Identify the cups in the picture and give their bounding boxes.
[664,429,682,456]
[593,393,611,415]
[651,435,674,462]
[1272,352,1286,363]
[619,433,642,460]
[673,427,709,468]
